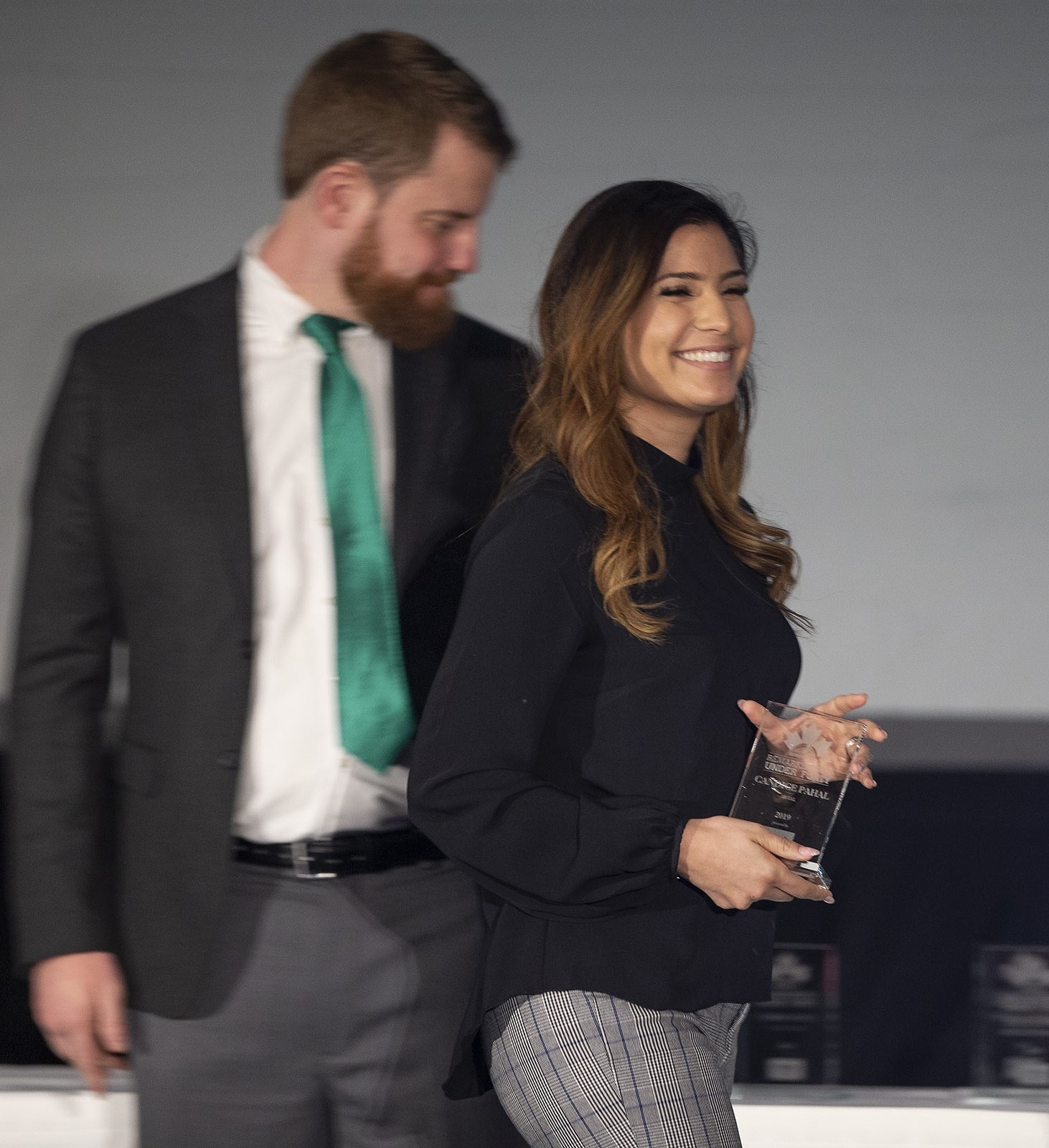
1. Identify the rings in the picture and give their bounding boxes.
[845,735,865,759]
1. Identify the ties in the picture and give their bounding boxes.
[300,312,415,774]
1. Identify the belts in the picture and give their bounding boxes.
[230,826,451,879]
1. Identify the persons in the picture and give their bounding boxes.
[405,181,887,1148]
[10,29,543,1148]
